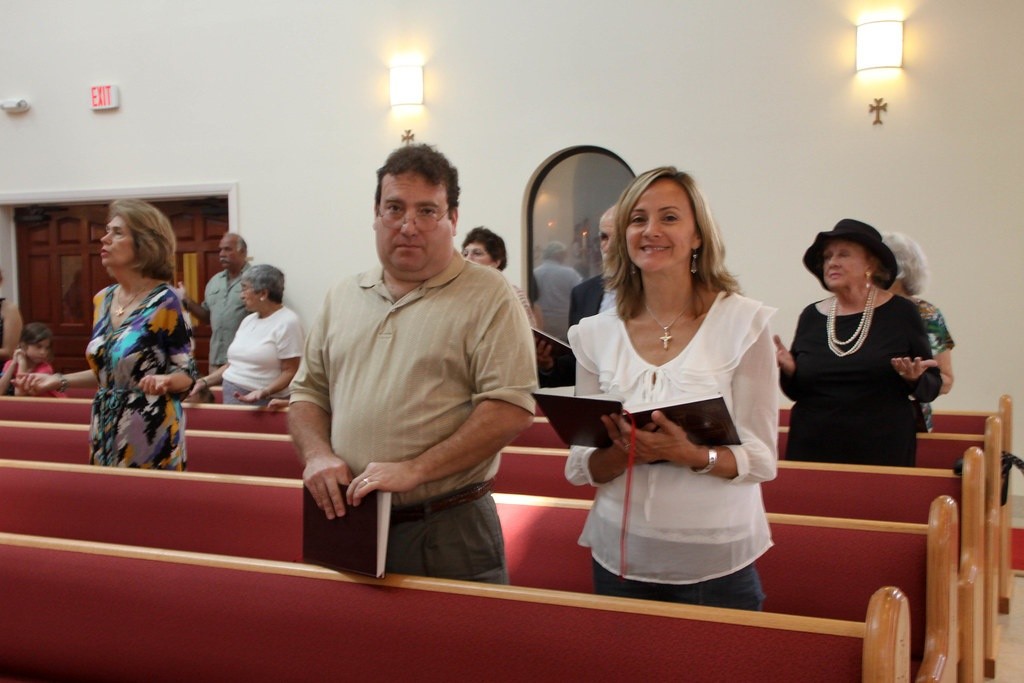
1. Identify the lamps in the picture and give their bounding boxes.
[855,20,904,73]
[389,65,424,109]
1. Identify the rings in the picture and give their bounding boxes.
[900,371,905,376]
[364,479,368,484]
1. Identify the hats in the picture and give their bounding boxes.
[803,219,899,291]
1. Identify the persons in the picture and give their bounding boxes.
[564,167,777,613]
[286,144,539,586]
[773,219,942,469]
[0,322,68,398]
[189,264,306,407]
[177,232,251,375]
[567,205,617,328]
[533,241,583,343]
[461,227,537,328]
[10,197,199,472]
[880,233,955,432]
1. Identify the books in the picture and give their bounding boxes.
[303,483,392,579]
[531,391,742,466]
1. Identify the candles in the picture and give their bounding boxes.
[582,231,588,250]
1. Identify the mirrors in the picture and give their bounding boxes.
[521,146,636,343]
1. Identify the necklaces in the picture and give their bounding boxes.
[645,304,689,349]
[826,285,879,357]
[116,287,145,317]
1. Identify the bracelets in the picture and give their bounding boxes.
[203,379,207,388]
[54,373,67,393]
[691,448,717,475]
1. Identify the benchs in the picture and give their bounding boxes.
[0,387,1012,682]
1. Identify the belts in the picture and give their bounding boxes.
[392,478,496,519]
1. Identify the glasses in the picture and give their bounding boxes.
[377,206,448,232]
